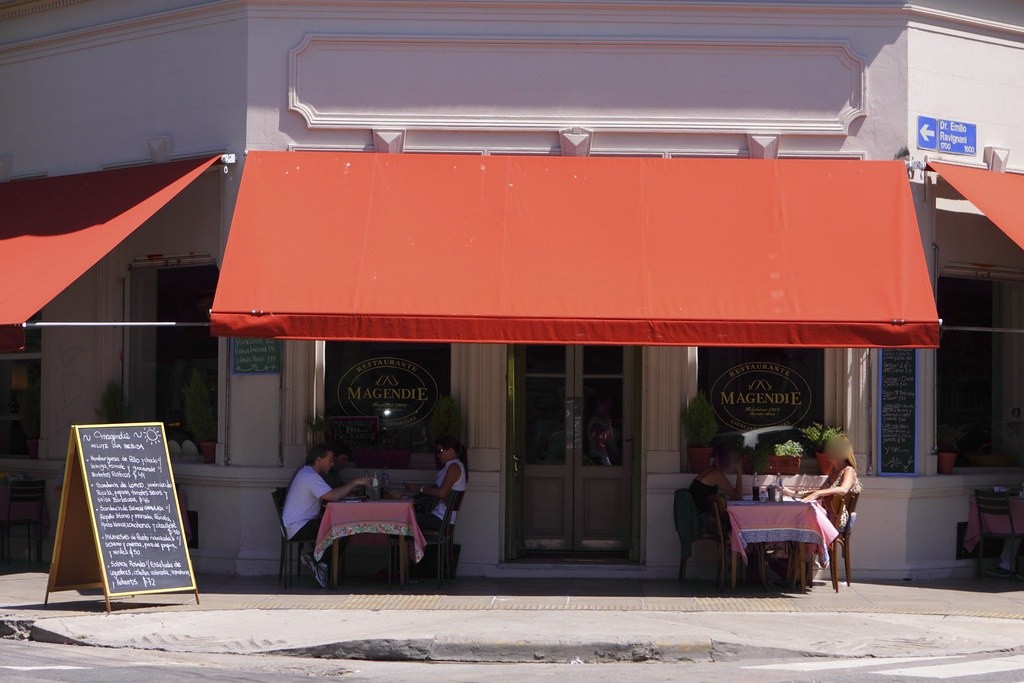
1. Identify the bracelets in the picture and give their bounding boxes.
[420,487,424,493]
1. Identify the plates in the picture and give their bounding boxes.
[182,440,198,456]
[342,495,367,500]
[168,439,182,458]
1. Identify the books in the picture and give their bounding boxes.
[338,496,368,503]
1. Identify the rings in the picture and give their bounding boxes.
[368,481,370,484]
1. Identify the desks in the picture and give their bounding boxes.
[0,486,51,539]
[962,495,1024,552]
[724,498,840,594]
[314,494,427,590]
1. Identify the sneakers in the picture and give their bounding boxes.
[300,551,329,588]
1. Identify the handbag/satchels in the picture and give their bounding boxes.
[413,482,441,512]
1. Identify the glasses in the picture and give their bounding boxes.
[434,446,447,455]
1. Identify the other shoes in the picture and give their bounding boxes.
[391,572,418,584]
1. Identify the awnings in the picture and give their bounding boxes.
[927,161,1024,250]
[208,151,943,347]
[0,152,226,327]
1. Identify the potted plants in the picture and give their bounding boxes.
[737,446,759,471]
[17,378,40,459]
[937,424,966,474]
[182,369,217,463]
[773,440,804,474]
[683,392,716,474]
[799,421,841,474]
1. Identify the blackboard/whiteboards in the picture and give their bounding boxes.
[877,347,920,476]
[47,423,196,597]
[230,336,281,375]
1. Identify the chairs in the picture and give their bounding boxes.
[268,486,342,590]
[673,487,859,591]
[972,486,1024,582]
[386,488,465,589]
[0,479,46,566]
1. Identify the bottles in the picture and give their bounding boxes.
[752,472,760,501]
[364,465,389,500]
[760,491,768,502]
[768,484,774,500]
[775,473,783,502]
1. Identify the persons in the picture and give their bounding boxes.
[388,435,466,586]
[992,538,1021,574]
[773,434,864,588]
[689,434,769,587]
[280,444,372,589]
[586,390,621,467]
